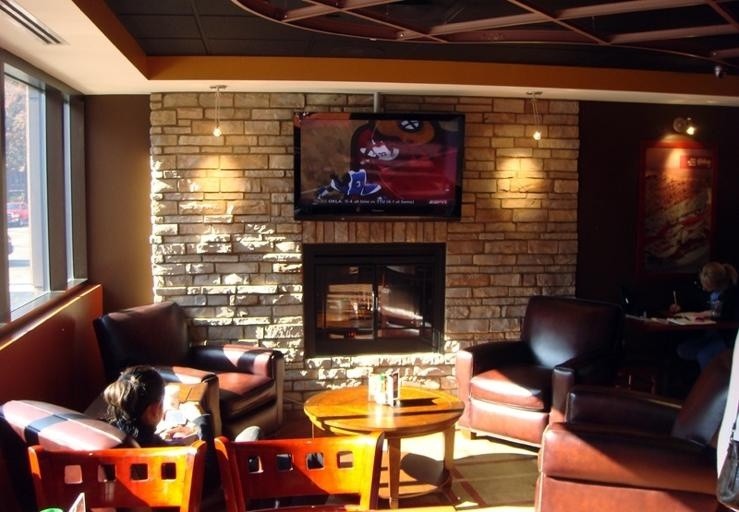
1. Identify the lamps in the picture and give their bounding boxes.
[211,85,227,139]
[527,92,542,142]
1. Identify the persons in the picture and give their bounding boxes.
[669,261,738,370]
[97,364,266,512]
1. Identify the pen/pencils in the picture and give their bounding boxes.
[672,291,678,305]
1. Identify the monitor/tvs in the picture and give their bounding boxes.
[293,112,465,220]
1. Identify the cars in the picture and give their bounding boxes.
[1,202,28,228]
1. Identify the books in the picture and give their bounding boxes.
[653,315,717,327]
